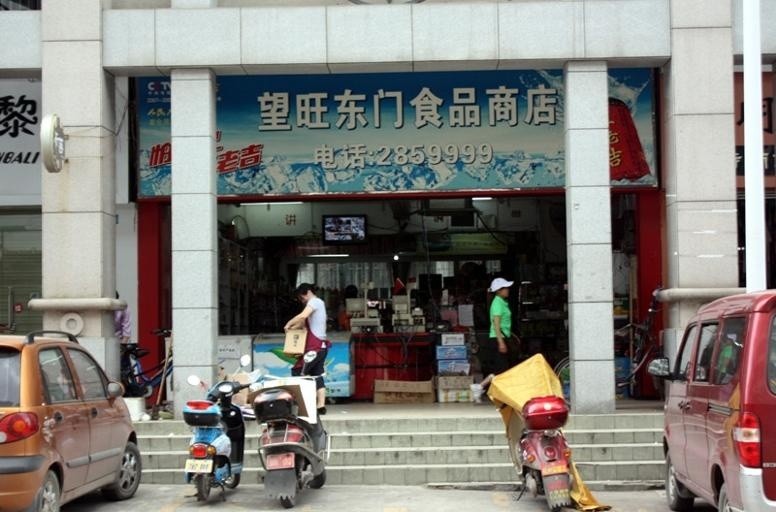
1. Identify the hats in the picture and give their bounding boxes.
[293,282,315,294]
[487,278,514,293]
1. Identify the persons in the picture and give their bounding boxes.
[470,278,514,404]
[283,283,328,415]
[114,290,131,344]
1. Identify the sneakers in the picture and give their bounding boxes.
[469,382,487,406]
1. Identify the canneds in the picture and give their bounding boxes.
[609,97,650,181]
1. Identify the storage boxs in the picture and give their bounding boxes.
[373,379,436,405]
[436,333,483,402]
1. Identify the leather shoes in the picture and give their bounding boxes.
[318,407,327,415]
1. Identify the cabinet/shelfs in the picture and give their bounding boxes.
[219,236,249,334]
[352,333,428,401]
[520,282,565,362]
[253,290,303,333]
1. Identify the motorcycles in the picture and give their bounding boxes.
[485,352,572,511]
[182,354,252,502]
[246,350,327,509]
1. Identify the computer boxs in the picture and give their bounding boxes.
[351,326,383,335]
[393,326,425,334]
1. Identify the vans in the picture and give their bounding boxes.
[645,289,775,512]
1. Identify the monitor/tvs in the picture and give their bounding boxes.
[391,295,412,311]
[448,213,478,231]
[345,298,368,316]
[322,213,368,246]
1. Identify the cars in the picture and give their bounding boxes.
[0,330,140,512]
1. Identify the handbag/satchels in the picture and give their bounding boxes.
[505,338,530,365]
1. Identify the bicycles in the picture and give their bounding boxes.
[553,303,663,406]
[120,326,172,399]
